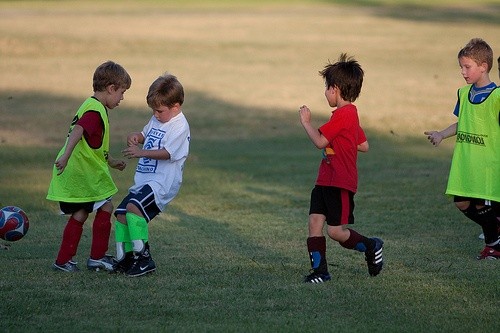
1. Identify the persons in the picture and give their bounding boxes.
[111,74,191,277]
[298,52,385,284]
[424,37,500,260]
[46,60,132,274]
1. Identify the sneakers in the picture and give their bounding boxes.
[304,270,331,284]
[112,243,157,277]
[87,253,116,273]
[52,260,83,274]
[477,244,500,261]
[365,237,384,277]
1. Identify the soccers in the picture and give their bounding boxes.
[0,205,30,242]
